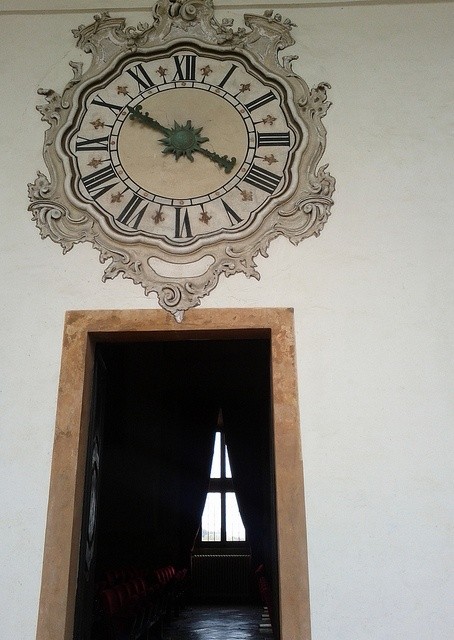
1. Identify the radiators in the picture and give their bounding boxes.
[191,555,252,599]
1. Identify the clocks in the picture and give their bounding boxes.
[26,1,336,321]
